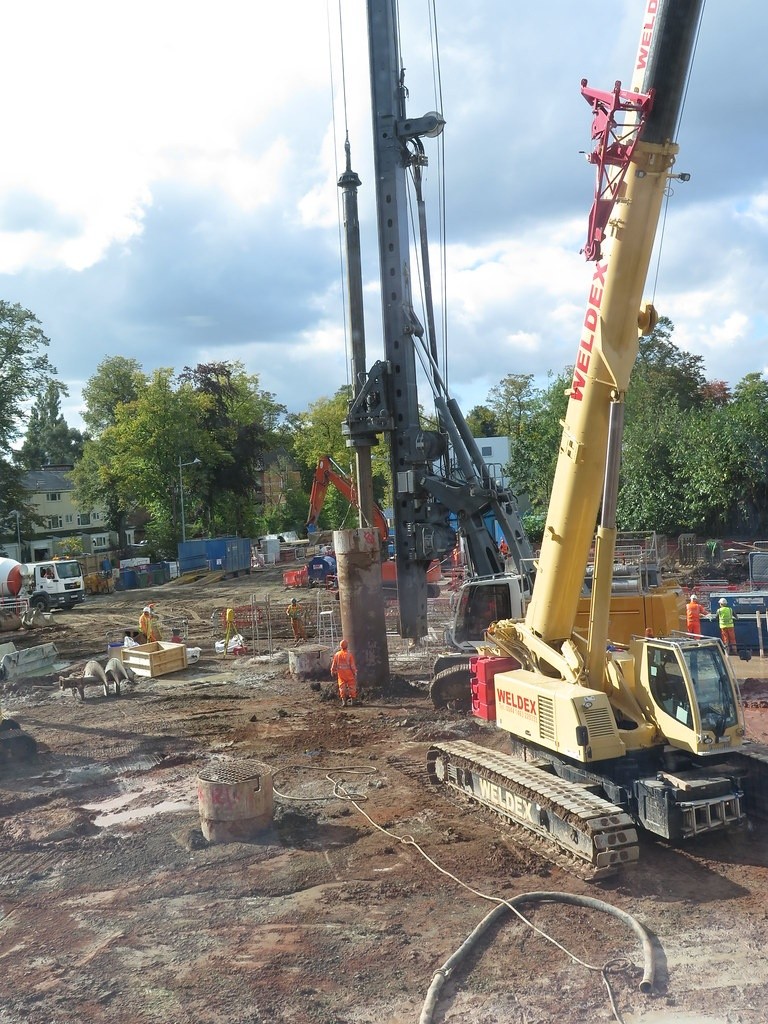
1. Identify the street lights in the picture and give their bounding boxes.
[179,456,202,542]
[11,510,22,564]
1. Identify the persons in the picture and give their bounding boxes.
[710,598,738,655]
[330,639,358,707]
[138,605,161,642]
[286,598,303,638]
[686,595,708,640]
[500,539,508,555]
[43,568,53,577]
[53,554,59,560]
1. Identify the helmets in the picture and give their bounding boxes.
[143,607,150,613]
[690,594,698,600]
[718,598,727,604]
[340,641,348,648]
[291,598,297,603]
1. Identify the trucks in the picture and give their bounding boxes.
[0,557,88,615]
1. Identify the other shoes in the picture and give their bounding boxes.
[350,698,357,705]
[341,700,347,706]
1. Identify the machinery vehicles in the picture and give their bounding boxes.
[305,454,442,602]
[336,0,685,715]
[424,0,755,881]
